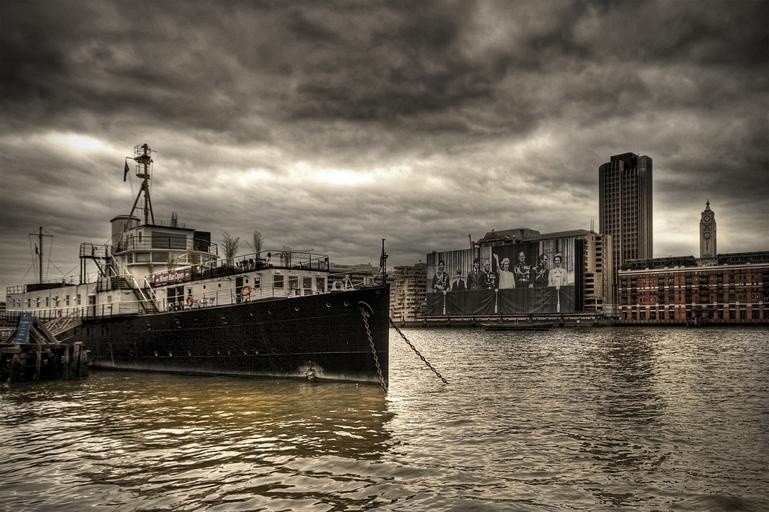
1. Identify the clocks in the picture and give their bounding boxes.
[704,216,710,221]
[704,232,711,238]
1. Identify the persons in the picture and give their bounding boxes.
[431,250,568,293]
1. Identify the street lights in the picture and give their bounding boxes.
[442,289,448,314]
[494,288,499,314]
[555,284,561,312]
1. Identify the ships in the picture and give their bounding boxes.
[0,140,397,391]
